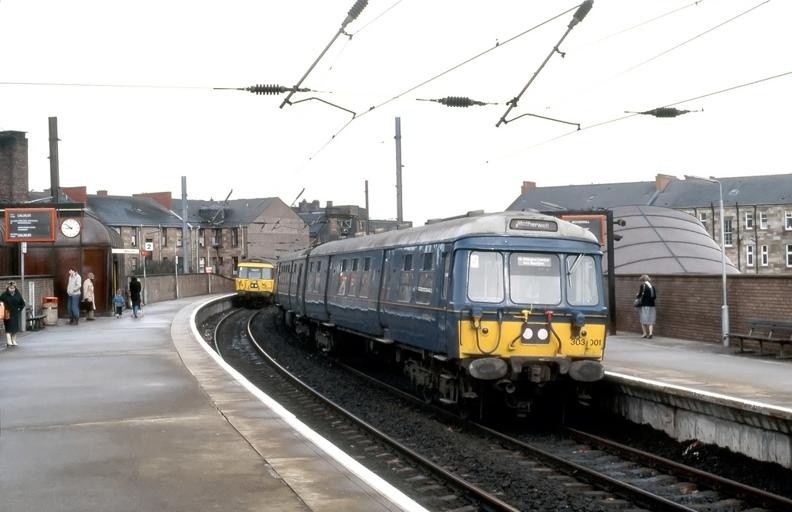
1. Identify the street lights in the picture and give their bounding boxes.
[683,174,729,348]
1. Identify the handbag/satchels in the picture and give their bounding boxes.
[633,297,642,307]
[79,300,92,311]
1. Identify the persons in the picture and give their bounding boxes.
[83,272,97,320]
[130,278,141,318]
[112,289,125,318]
[635,274,657,339]
[0,281,26,346]
[66,268,81,325]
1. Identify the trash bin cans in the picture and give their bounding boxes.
[43,296,59,326]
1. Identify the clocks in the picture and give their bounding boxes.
[60,218,80,238]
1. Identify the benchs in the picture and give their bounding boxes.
[26,305,47,331]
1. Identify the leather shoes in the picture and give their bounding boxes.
[641,333,652,338]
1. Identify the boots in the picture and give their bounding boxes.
[6,332,17,346]
